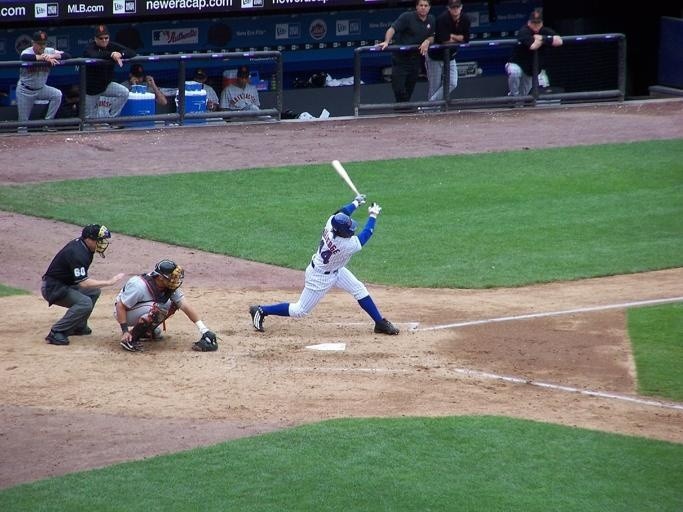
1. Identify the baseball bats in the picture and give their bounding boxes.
[331,158,359,194]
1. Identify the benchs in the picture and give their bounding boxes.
[0,75,564,133]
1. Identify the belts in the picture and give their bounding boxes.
[22,83,40,91]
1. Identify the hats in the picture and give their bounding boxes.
[194,68,208,79]
[237,65,249,78]
[448,0,461,7]
[32,30,49,44]
[95,25,110,37]
[131,63,145,78]
[530,11,542,23]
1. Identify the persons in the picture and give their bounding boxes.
[113,259,216,352]
[373,2,472,113]
[16,26,271,132]
[249,194,399,335]
[41,224,124,345]
[505,11,562,109]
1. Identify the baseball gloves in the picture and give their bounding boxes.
[193,330,217,351]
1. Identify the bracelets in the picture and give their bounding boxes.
[121,323,128,334]
[196,320,209,334]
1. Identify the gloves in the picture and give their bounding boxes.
[355,194,368,207]
[367,202,382,216]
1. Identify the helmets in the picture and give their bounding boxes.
[80,223,111,258]
[150,259,184,292]
[331,212,359,233]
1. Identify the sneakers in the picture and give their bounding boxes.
[46,326,92,345]
[249,305,266,332]
[120,339,137,352]
[374,317,399,334]
[140,331,163,342]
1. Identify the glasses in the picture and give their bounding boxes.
[98,37,108,40]
